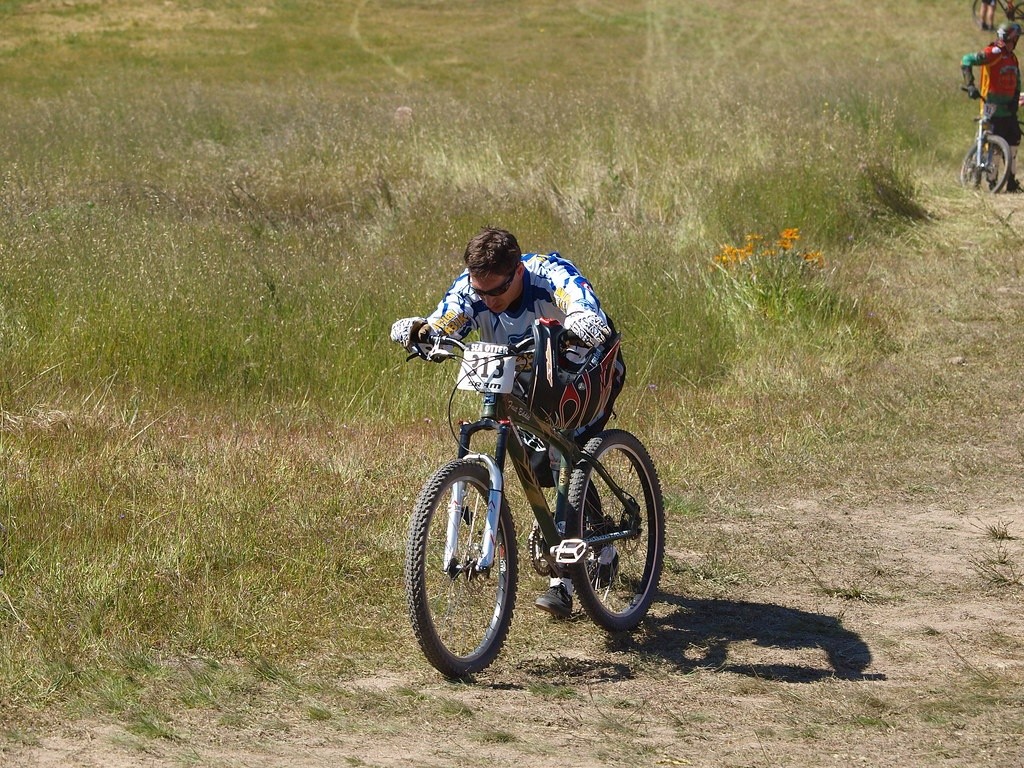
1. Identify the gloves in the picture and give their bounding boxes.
[967,85,979,100]
[564,312,611,348]
[390,317,432,352]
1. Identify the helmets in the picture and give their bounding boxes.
[996,22,1021,41]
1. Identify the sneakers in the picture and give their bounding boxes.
[536,584,572,618]
[586,551,619,588]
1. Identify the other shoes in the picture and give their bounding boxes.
[979,22,988,29]
[986,25,995,32]
[989,183,996,191]
[1007,182,1023,193]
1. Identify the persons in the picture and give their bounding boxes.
[980,0,996,32]
[390,227,627,617]
[960,21,1024,193]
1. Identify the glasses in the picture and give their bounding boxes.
[468,270,516,296]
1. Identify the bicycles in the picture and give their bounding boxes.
[973,0,1024,36]
[396,315,667,678]
[958,84,1024,195]
